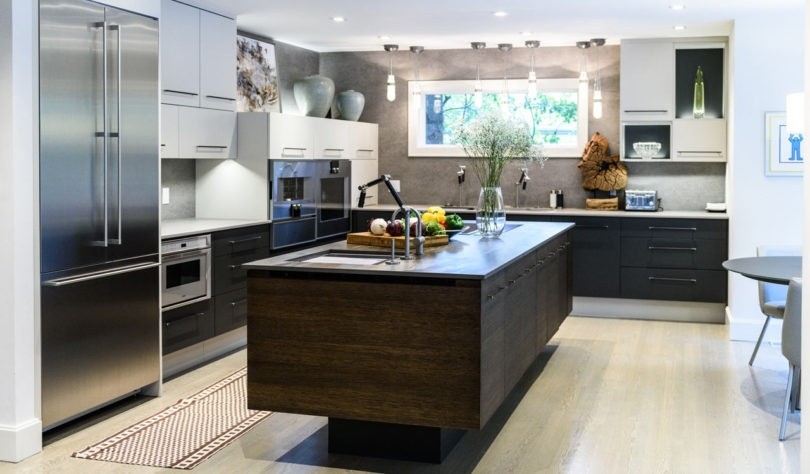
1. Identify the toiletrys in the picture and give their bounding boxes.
[557,190,564,209]
[549,189,557,209]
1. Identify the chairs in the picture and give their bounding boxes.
[778,277,800,441]
[747,245,801,367]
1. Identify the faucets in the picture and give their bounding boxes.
[357,173,413,259]
[390,206,425,258]
[457,166,468,208]
[513,168,532,209]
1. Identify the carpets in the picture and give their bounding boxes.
[71,367,276,470]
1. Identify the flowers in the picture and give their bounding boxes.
[455,118,552,234]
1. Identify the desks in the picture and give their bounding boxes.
[721,254,800,411]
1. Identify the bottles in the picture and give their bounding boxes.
[557,190,563,210]
[549,190,558,209]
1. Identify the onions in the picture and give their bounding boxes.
[368,217,425,236]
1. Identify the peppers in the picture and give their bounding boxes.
[401,205,464,235]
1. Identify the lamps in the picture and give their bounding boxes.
[786,91,808,138]
[592,47,605,118]
[473,50,484,109]
[578,46,588,109]
[384,50,398,102]
[525,48,537,97]
[500,50,510,121]
[413,50,424,118]
[433,93,442,116]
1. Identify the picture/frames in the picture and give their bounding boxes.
[763,109,805,179]
[236,33,282,112]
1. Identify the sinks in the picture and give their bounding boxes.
[440,205,476,212]
[505,207,554,212]
[283,247,414,267]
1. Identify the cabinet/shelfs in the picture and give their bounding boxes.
[246,222,575,435]
[621,38,726,163]
[569,216,728,307]
[195,111,381,223]
[158,0,240,161]
[159,225,271,376]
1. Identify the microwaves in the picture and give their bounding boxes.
[161,255,207,309]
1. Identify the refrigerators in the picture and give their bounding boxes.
[38,0,163,431]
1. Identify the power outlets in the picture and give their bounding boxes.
[388,179,400,193]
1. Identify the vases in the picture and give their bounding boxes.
[292,71,336,118]
[475,185,505,237]
[335,90,366,122]
[692,64,706,119]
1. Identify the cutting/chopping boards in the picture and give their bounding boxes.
[347,231,449,247]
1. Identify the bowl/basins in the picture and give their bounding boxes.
[633,143,662,160]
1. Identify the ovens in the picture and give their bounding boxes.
[269,159,352,250]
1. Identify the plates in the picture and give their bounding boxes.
[705,202,726,211]
[444,230,461,239]
